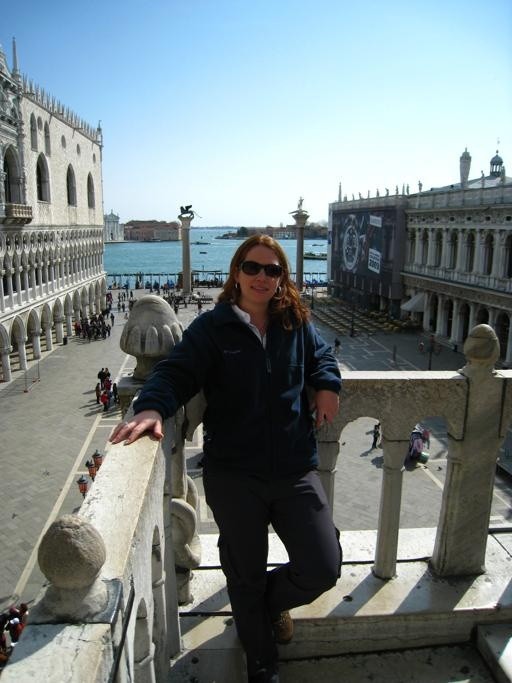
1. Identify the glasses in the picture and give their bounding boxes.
[242,259,282,277]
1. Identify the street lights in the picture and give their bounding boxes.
[418,334,443,370]
[77,449,103,500]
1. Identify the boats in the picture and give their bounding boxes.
[190,240,211,244]
[140,239,160,241]
[304,251,327,259]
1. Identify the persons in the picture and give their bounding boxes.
[0,604,29,675]
[334,338,341,355]
[371,423,380,449]
[74,277,218,343]
[109,233,342,683]
[96,368,120,412]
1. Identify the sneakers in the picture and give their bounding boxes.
[269,609,294,646]
